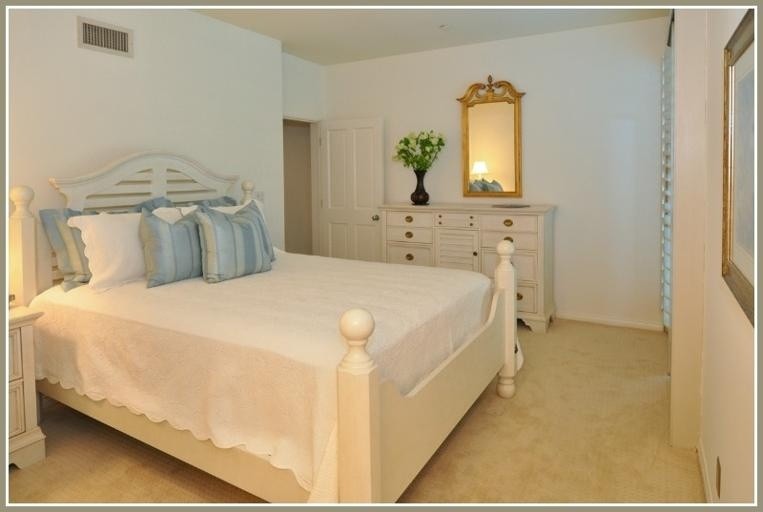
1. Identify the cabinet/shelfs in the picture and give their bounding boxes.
[376,201,557,335]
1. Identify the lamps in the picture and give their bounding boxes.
[468,158,488,179]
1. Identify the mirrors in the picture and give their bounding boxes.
[453,73,526,199]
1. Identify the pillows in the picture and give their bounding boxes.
[37,186,275,291]
[470,178,503,192]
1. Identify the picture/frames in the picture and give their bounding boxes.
[717,8,757,329]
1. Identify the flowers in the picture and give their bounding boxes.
[392,131,446,169]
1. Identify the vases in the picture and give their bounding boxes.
[409,166,431,206]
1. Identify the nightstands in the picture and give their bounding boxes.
[9,303,48,474]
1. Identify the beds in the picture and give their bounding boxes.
[7,149,533,506]
